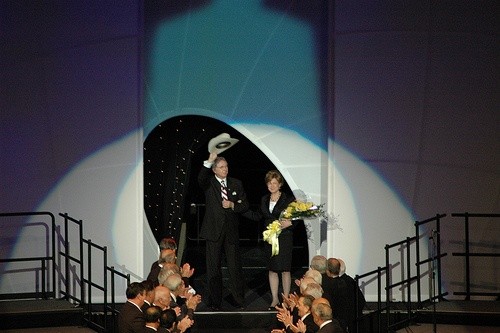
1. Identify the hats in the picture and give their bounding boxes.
[208,134,238,155]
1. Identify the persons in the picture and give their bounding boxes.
[119,238,201,333]
[265,258,367,333]
[198,148,249,312]
[260,170,296,311]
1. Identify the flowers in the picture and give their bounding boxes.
[280,201,327,220]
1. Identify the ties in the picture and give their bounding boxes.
[221,181,228,201]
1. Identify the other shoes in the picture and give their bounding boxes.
[233,298,240,305]
[268,302,281,311]
[211,304,220,311]
